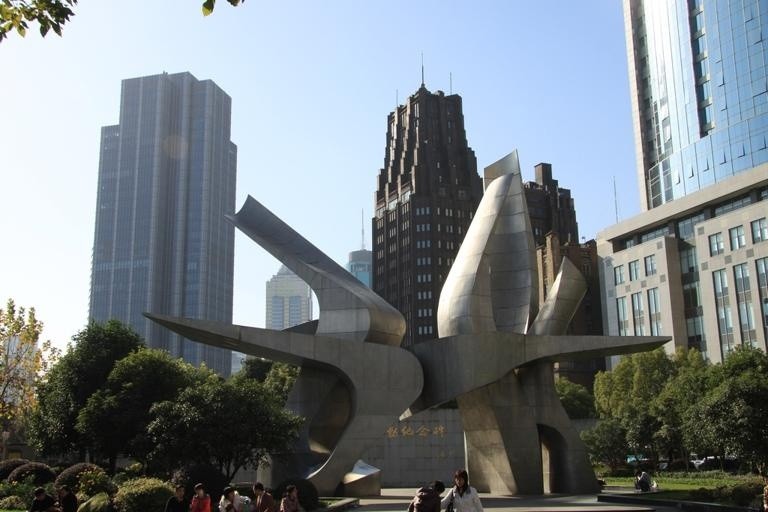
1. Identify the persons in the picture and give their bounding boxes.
[633,468,651,491]
[251,481,275,512]
[279,483,305,512]
[440,468,483,512]
[29,484,54,512]
[763,475,768,512]
[51,485,78,512]
[406,480,446,512]
[190,482,212,512]
[218,486,252,512]
[164,483,189,512]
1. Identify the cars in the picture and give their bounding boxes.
[625,454,745,472]
